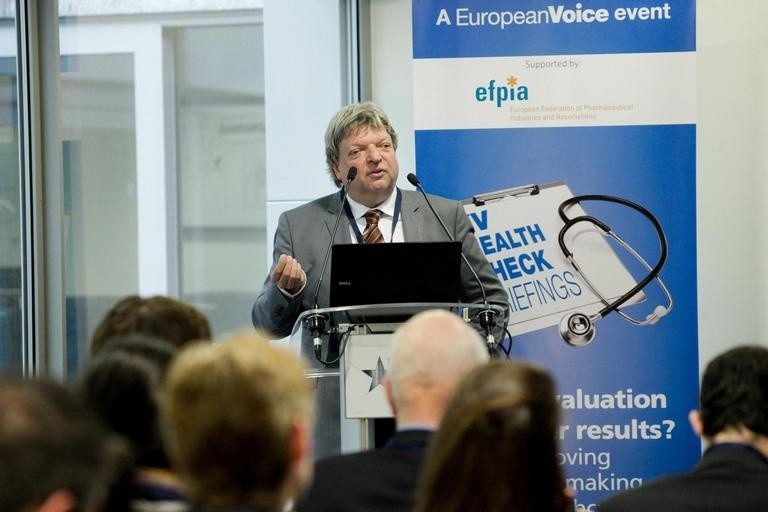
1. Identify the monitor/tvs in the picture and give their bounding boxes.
[328,241,463,307]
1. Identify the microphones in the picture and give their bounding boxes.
[407,173,487,304]
[313,166,358,306]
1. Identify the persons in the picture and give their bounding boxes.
[297,308,491,512]
[78,333,177,470]
[590,341,767,512]
[250,99,510,466]
[1,367,132,512]
[154,332,319,512]
[414,354,582,512]
[88,292,212,381]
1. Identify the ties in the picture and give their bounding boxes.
[360,209,386,244]
